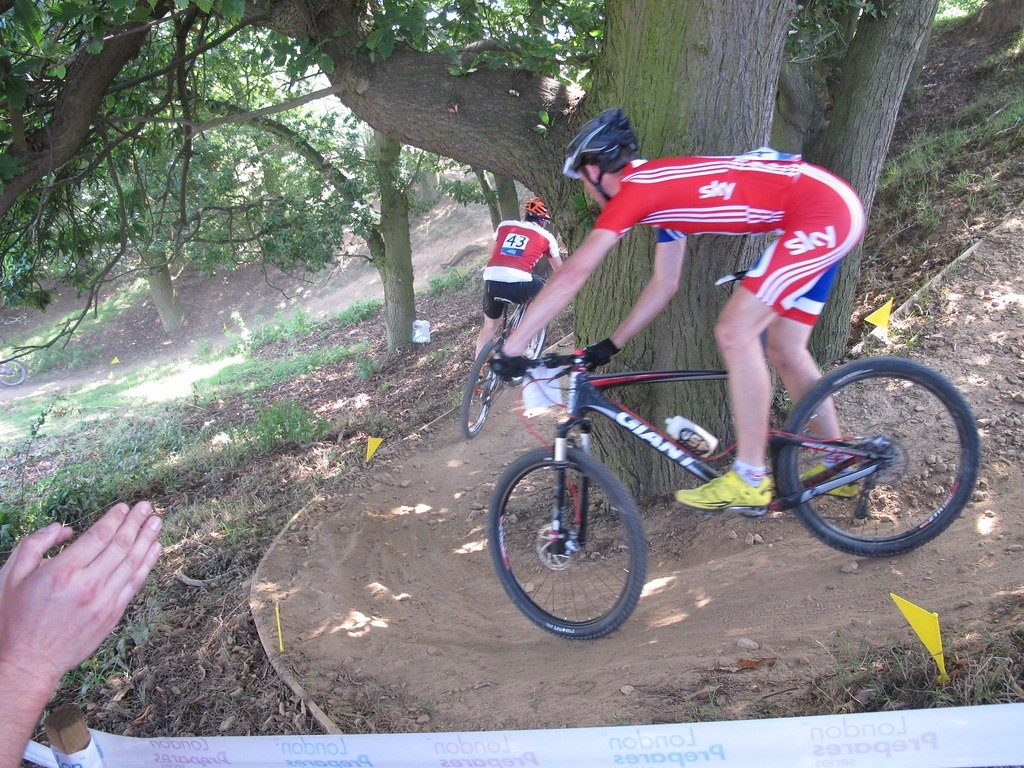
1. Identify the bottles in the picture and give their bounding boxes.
[665,415,719,459]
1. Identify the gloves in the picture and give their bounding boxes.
[580,337,621,373]
[490,354,525,383]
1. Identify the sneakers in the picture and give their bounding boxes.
[799,465,860,497]
[674,471,772,510]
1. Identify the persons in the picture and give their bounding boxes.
[474,197,563,394]
[491,109,868,517]
[0,501,162,768]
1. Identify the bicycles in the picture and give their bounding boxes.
[459,297,548,441]
[485,339,981,641]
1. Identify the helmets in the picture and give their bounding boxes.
[525,197,551,220]
[562,109,637,178]
